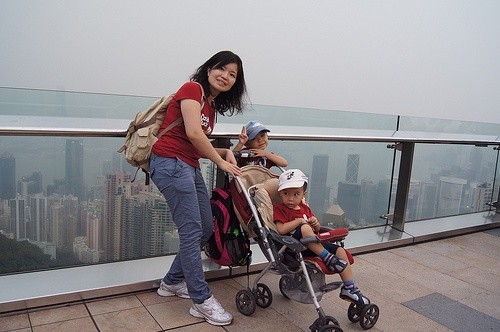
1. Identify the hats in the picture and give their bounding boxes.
[245,120,271,141]
[278,169,308,191]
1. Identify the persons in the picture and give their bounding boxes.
[149,50,245,326]
[232,121,288,167]
[273,169,370,305]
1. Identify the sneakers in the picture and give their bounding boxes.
[338,284,370,306]
[157,280,191,299]
[190,294,233,325]
[322,253,348,273]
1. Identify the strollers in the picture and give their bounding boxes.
[228,151,380,332]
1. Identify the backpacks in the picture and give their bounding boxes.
[117,81,206,171]
[204,173,252,266]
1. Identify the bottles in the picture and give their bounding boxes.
[226,226,238,237]
